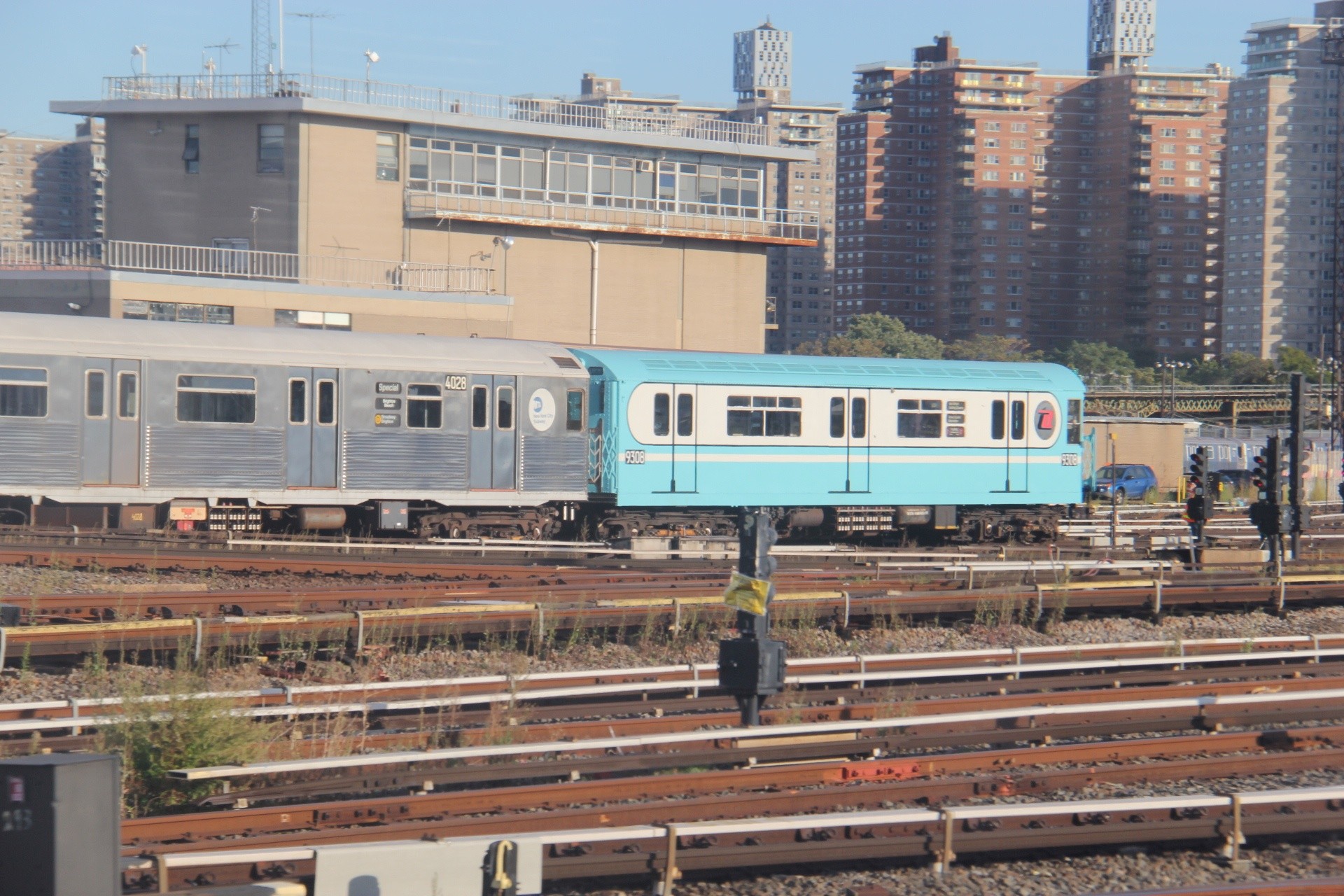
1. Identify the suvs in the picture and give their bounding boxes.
[1083,463,1157,505]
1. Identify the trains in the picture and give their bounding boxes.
[0,311,1097,539]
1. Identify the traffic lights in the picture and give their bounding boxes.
[1187,497,1207,519]
[1188,445,1209,496]
[1275,435,1291,504]
[1288,452,1311,501]
[1291,373,1313,430]
[1252,436,1276,503]
[737,509,778,634]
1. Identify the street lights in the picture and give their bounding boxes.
[1155,357,1192,416]
